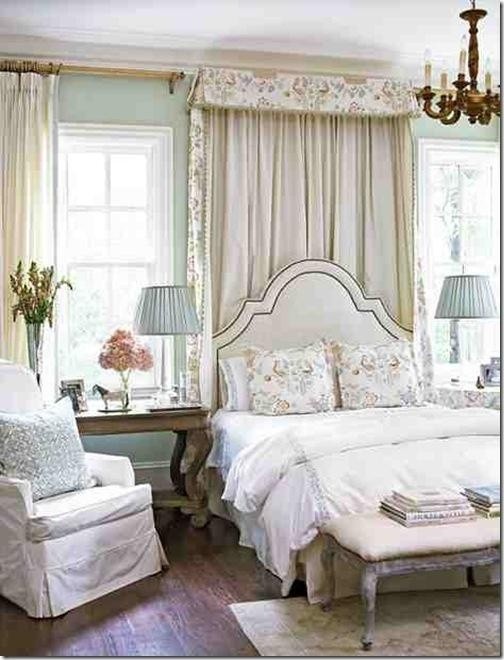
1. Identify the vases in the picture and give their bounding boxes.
[118,378,130,411]
[26,322,40,377]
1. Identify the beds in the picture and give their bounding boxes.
[204,258,500,596]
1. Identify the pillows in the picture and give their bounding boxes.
[0,394,99,503]
[218,349,251,414]
[332,338,426,408]
[244,336,335,415]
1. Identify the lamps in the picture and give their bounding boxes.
[433,275,500,319]
[414,0,502,126]
[131,285,201,407]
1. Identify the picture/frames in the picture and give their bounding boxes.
[481,364,501,386]
[61,379,89,412]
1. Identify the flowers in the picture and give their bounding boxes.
[97,327,153,404]
[8,258,73,366]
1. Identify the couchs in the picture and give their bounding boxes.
[1,360,170,625]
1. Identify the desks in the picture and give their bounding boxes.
[65,398,212,532]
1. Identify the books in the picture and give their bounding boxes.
[378,486,501,527]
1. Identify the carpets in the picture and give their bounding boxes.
[227,584,501,658]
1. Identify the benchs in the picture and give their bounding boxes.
[319,507,500,648]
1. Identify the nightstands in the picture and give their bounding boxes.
[434,386,500,410]
